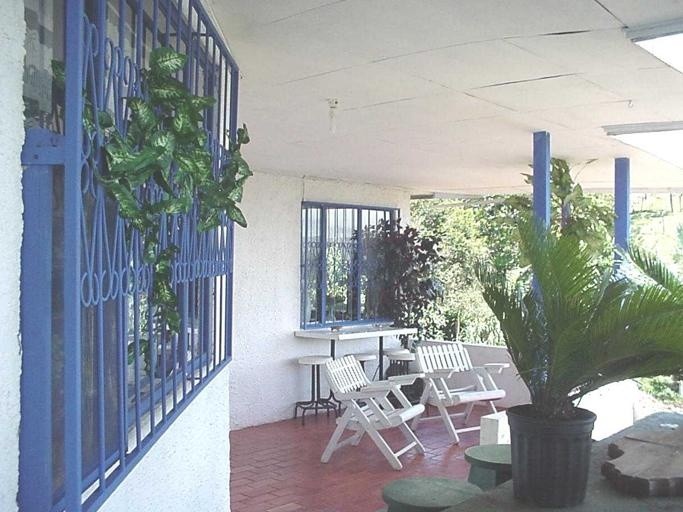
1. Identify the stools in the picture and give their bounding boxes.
[294,355,338,422]
[464,444,514,488]
[380,478,483,510]
[346,354,377,410]
[391,353,416,378]
[385,348,412,386]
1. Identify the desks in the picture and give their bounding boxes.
[294,326,419,416]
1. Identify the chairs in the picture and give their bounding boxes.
[320,354,428,472]
[416,342,511,445]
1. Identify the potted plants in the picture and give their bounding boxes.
[481,198,683,508]
